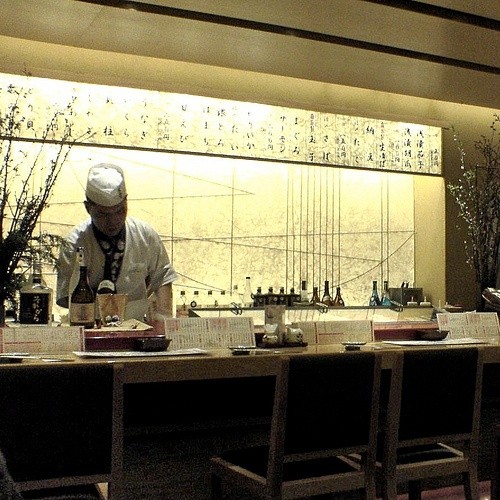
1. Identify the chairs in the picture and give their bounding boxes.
[210,347,485,500]
[0,364,125,500]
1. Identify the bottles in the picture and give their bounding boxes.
[369,281,380,306]
[334,287,344,306]
[310,287,320,306]
[18,259,54,326]
[176,276,294,318]
[380,281,392,306]
[322,281,333,306]
[68,244,118,329]
[300,280,309,303]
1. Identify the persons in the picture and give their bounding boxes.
[56,162,178,324]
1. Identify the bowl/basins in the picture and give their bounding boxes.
[416,330,449,341]
[137,338,172,352]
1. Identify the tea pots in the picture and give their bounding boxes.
[285,323,304,342]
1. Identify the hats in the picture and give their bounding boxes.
[86,163,128,207]
